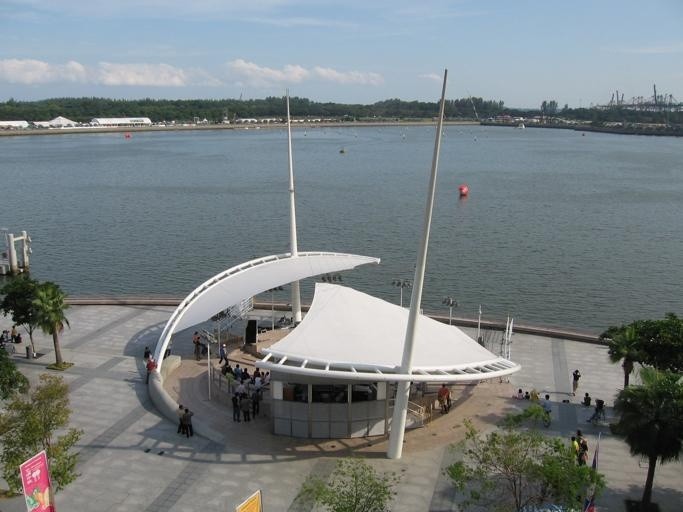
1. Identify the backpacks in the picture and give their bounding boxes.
[577,437,589,452]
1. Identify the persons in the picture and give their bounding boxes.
[571,369,582,394]
[142,346,153,367]
[191,330,271,422]
[163,338,172,358]
[176,404,185,433]
[580,392,591,406]
[569,429,590,467]
[438,383,449,414]
[145,355,156,384]
[541,394,551,413]
[584,398,604,422]
[0,325,21,344]
[524,391,529,400]
[517,388,523,399]
[181,409,193,437]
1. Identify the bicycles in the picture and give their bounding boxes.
[540,409,552,427]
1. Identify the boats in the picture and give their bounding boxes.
[516,123,524,129]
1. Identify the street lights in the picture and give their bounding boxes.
[390,276,413,309]
[442,297,459,324]
[320,274,342,284]
[265,285,284,331]
[197,326,217,401]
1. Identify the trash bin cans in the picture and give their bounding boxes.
[26,345,33,359]
